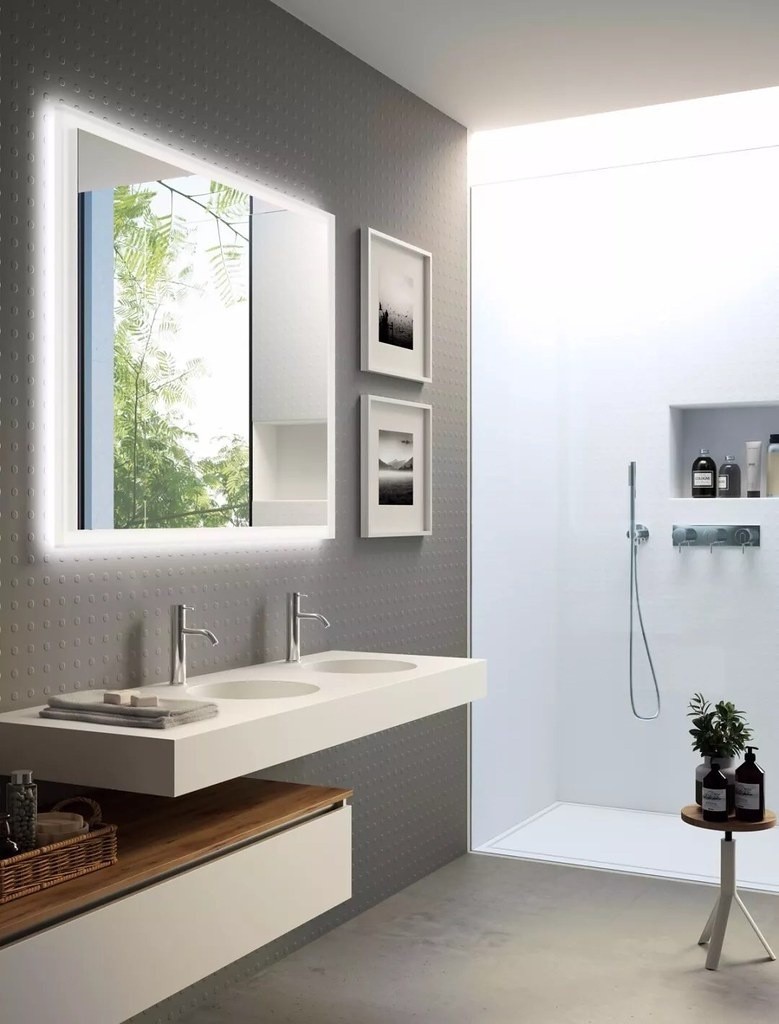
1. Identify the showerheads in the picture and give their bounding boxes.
[626,460,640,540]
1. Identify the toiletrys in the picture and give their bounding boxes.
[690,447,718,499]
[764,433,779,498]
[732,744,768,823]
[701,761,733,823]
[717,455,742,498]
[743,439,764,498]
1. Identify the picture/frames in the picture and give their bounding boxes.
[360,394,432,538]
[360,224,433,385]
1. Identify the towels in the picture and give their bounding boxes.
[37,688,220,730]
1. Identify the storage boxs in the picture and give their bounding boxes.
[0,797,118,903]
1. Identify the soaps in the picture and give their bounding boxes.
[103,688,143,705]
[129,693,160,708]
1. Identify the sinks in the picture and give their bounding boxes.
[187,680,322,701]
[307,657,421,675]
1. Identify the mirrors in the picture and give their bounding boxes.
[77,127,336,532]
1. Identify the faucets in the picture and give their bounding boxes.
[168,603,219,686]
[285,590,332,663]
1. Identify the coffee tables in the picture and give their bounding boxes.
[681,805,777,972]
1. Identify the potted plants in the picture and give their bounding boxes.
[686,693,754,807]
